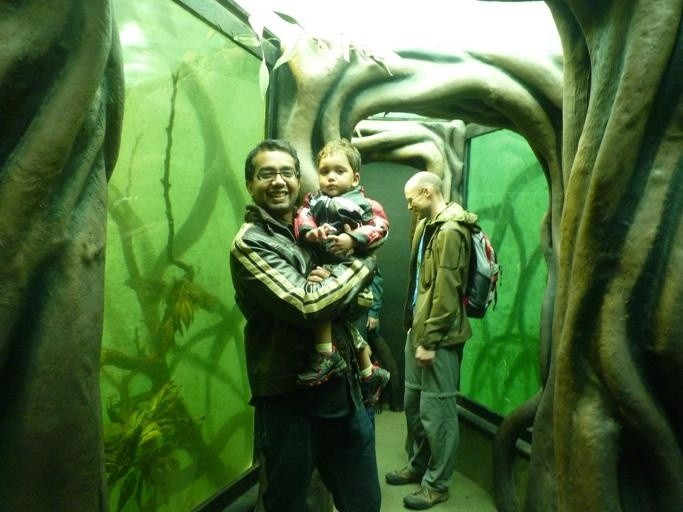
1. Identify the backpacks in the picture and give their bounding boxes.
[437,222,499,319]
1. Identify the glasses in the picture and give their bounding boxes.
[257,167,297,181]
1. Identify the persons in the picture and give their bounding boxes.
[227,135,382,512]
[293,138,392,408]
[354,264,400,416]
[385,170,480,510]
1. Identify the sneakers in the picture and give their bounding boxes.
[295,346,347,386]
[386,462,421,484]
[358,364,390,408]
[404,488,448,509]
